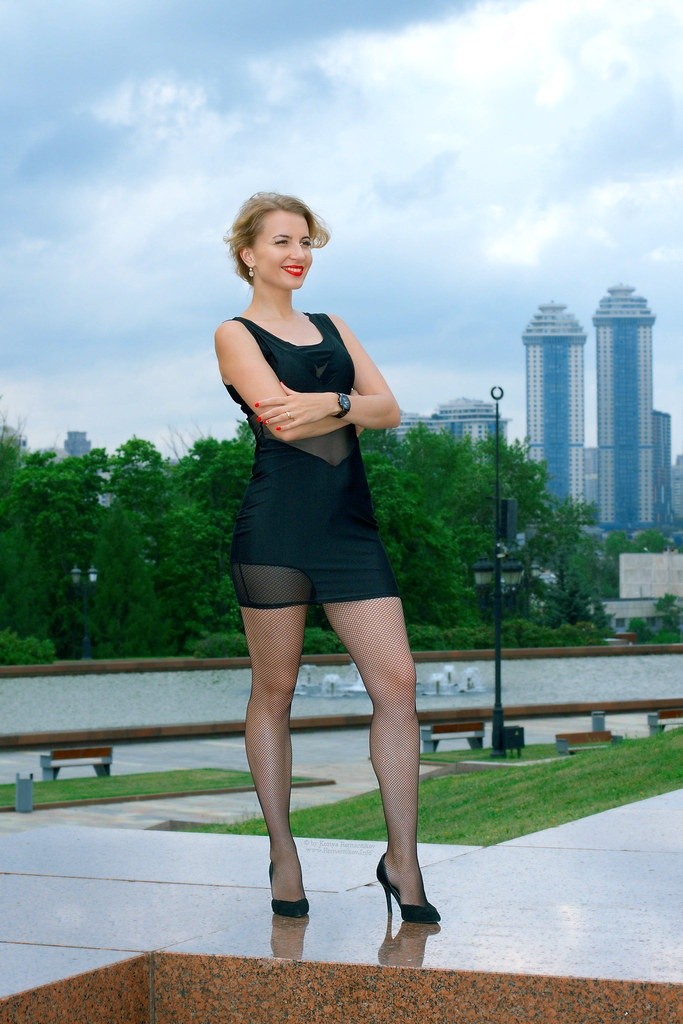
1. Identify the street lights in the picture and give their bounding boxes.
[490,386,506,758]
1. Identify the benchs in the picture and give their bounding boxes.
[40,748,113,780]
[648,709,683,736]
[556,731,623,756]
[421,722,485,752]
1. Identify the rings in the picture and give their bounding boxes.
[285,411,291,419]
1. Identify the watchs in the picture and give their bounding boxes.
[332,391,351,419]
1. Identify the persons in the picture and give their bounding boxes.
[214,194,445,926]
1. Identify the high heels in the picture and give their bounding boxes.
[269,862,309,917]
[377,853,441,923]
